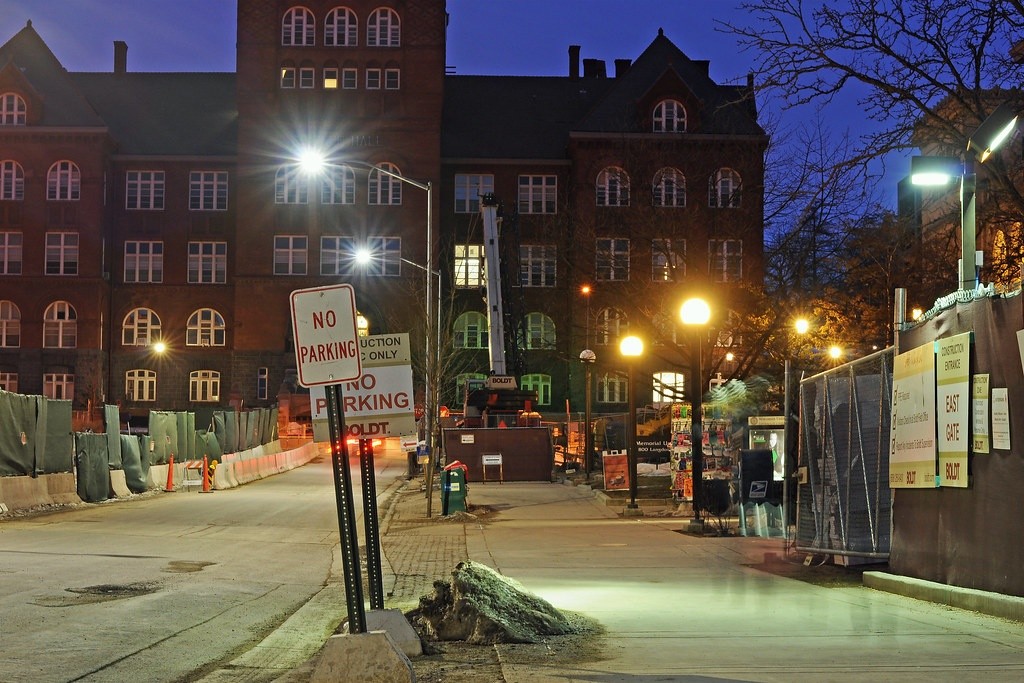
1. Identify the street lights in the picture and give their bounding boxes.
[580,349,597,469]
[297,146,435,481]
[350,246,442,422]
[581,286,591,350]
[910,103,1020,291]
[620,336,645,508]
[678,297,713,519]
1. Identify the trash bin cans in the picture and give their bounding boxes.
[737,448,774,503]
[438,468,467,515]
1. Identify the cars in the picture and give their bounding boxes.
[347,438,384,456]
